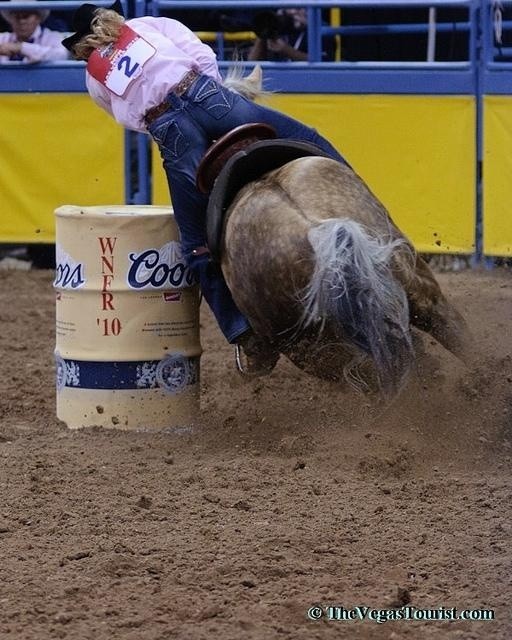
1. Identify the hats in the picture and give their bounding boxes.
[0,0,51,25]
[58,2,107,51]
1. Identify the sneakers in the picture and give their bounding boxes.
[242,335,281,375]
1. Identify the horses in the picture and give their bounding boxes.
[218,50,481,420]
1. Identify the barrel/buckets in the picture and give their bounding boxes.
[54,202,203,431]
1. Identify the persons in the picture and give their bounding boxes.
[247,9,332,62]
[2,0,95,66]
[59,4,356,376]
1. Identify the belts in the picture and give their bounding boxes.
[143,68,200,127]
